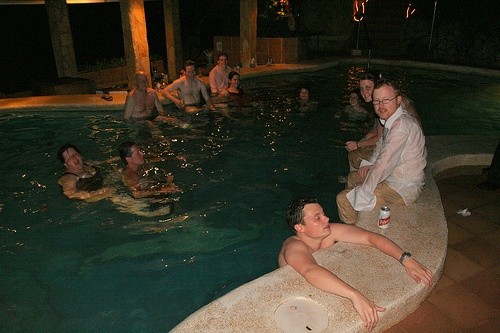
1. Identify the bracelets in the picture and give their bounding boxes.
[356,141,358,148]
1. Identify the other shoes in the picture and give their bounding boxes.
[479,181,500,191]
[482,167,491,175]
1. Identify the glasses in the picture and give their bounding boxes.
[373,96,398,104]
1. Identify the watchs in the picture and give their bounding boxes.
[399,251,411,264]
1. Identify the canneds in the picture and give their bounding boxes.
[378,206,391,229]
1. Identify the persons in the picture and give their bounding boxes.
[278,198,435,331]
[219,72,250,105]
[119,142,145,173]
[209,52,235,96]
[299,86,312,101]
[162,60,211,113]
[56,144,84,171]
[345,90,371,116]
[335,79,428,224]
[124,71,175,126]
[338,75,421,189]
